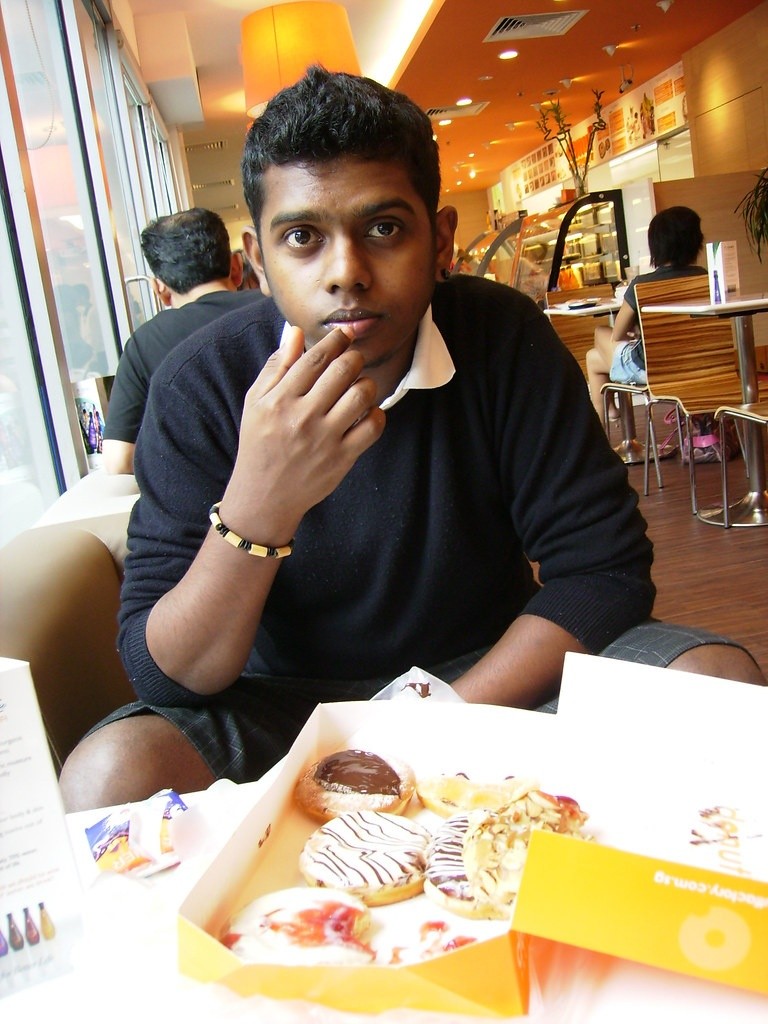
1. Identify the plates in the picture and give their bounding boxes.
[610,298,624,306]
[568,302,596,310]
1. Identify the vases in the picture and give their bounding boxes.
[573,174,588,198]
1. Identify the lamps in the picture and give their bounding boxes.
[242,1,361,119]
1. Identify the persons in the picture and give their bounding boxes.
[56,58,767,814]
[585,205,709,430]
[102,205,269,476]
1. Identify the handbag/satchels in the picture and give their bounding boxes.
[659,407,740,465]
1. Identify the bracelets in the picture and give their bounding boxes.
[209,500,297,559]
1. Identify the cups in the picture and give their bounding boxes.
[728,285,736,298]
[625,266,640,285]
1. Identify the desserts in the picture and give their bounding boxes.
[224,747,583,967]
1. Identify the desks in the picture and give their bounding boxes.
[641,294,768,527]
[543,299,672,465]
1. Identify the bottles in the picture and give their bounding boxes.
[714,270,721,304]
[558,266,580,290]
[0,902,57,956]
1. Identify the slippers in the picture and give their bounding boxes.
[607,411,621,422]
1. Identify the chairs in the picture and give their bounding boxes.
[0,468,139,770]
[545,275,768,528]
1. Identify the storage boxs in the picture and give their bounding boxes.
[174,651,768,1019]
[706,241,741,304]
